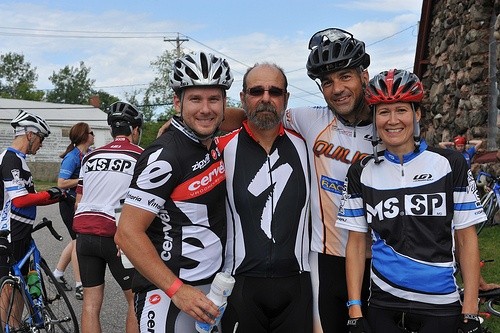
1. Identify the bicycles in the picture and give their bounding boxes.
[475,176,500,235]
[458,258,500,333]
[0,216,81,333]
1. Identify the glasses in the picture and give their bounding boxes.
[88,131,94,136]
[244,87,285,96]
[309,28,353,51]
[34,132,44,144]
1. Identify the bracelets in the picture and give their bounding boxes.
[346,299,362,308]
[165,278,182,298]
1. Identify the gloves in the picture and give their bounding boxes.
[345,317,369,333]
[59,189,70,202]
[47,186,61,199]
[457,314,481,333]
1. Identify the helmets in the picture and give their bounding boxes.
[364,69,424,105]
[306,37,370,80]
[169,51,233,94]
[10,110,50,137]
[107,101,143,128]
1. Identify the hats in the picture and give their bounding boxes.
[454,136,466,144]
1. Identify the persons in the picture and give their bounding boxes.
[155,27,432,333]
[0,111,73,333]
[213,60,316,333]
[448,213,500,333]
[437,135,483,193]
[76,101,158,333]
[47,121,96,300]
[113,50,254,333]
[339,68,487,333]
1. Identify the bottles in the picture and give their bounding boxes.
[195,268,235,333]
[27,265,41,300]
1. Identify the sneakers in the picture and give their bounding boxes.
[76,285,83,300]
[47,274,72,291]
